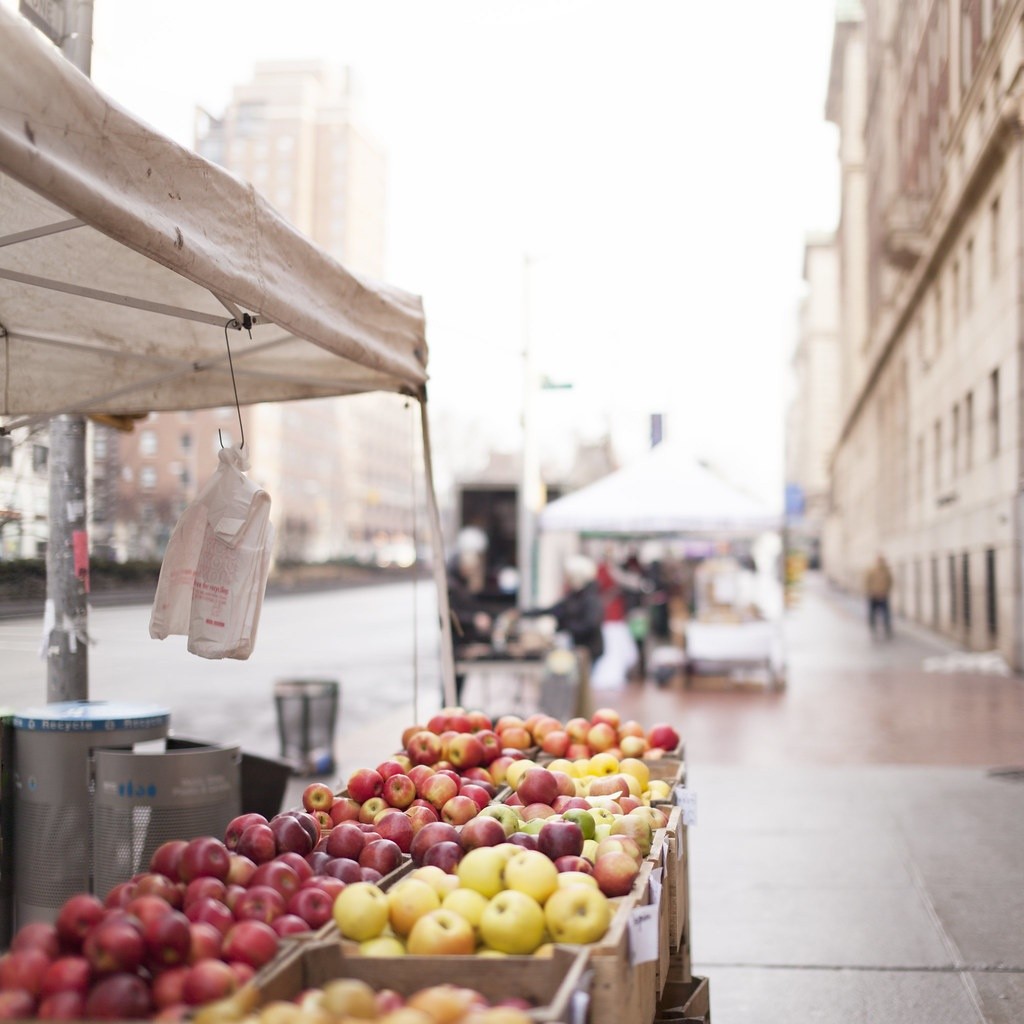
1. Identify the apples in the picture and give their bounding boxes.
[1,704,681,1024]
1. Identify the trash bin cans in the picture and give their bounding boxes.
[274,675,338,776]
[14,700,243,936]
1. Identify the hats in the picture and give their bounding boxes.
[565,555,598,584]
[453,529,487,554]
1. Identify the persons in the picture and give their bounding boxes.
[866,556,892,643]
[443,541,673,720]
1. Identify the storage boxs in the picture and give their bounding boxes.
[243,745,711,1024]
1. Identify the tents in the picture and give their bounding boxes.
[0,1,455,711]
[539,440,781,536]
